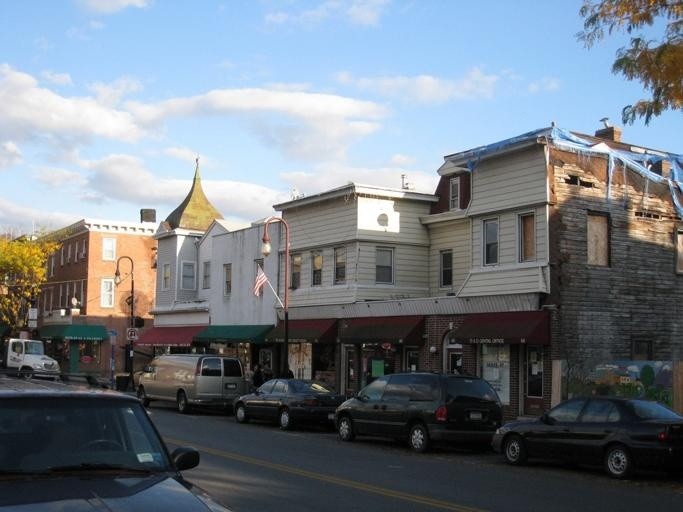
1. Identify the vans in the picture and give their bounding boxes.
[137,354,246,414]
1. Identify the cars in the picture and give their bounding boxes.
[490,395,683,485]
[231,377,347,431]
[0,376,236,512]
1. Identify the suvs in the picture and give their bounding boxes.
[333,371,504,453]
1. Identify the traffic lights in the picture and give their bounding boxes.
[133,316,144,328]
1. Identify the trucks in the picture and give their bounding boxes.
[0,338,61,380]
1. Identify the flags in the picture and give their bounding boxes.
[251,264,271,298]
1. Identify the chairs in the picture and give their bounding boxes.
[576,404,586,422]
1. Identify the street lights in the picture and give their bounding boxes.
[262,216,289,377]
[115,256,134,375]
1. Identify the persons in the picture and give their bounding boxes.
[253,364,264,387]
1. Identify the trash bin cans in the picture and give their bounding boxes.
[116,375,129,392]
